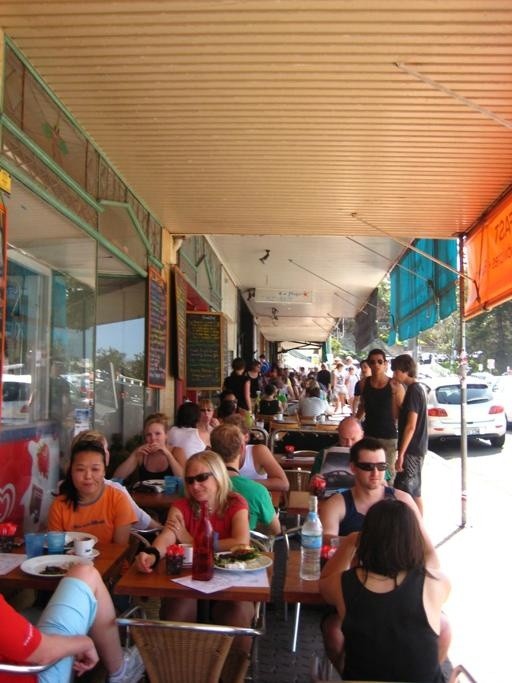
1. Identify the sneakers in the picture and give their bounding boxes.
[108,646,145,683]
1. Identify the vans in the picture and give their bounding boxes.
[1,374,73,436]
[96,379,142,407]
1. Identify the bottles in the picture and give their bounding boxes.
[191,500,215,580]
[299,510,323,582]
[308,494,323,529]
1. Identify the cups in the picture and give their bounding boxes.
[164,476,178,494]
[73,536,95,554]
[47,530,66,554]
[273,413,283,420]
[177,543,193,563]
[23,532,44,557]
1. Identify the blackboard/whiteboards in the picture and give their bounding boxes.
[184,312,224,391]
[146,267,170,390]
[172,264,187,382]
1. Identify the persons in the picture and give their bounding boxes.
[306,416,394,494]
[355,359,362,391]
[167,401,210,460]
[194,397,220,445]
[285,386,306,401]
[255,385,284,414]
[71,429,163,531]
[220,356,252,411]
[298,386,334,417]
[178,423,281,536]
[318,437,438,679]
[354,349,405,486]
[223,413,289,492]
[317,498,452,683]
[133,449,257,683]
[48,439,139,545]
[111,412,189,497]
[260,353,333,386]
[27,358,73,423]
[333,355,354,416]
[245,359,261,410]
[1,563,146,681]
[352,358,371,414]
[215,391,254,426]
[389,354,429,518]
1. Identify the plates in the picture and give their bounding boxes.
[66,547,100,557]
[40,530,99,549]
[20,553,94,577]
[213,549,274,571]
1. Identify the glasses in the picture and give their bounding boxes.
[369,360,383,364]
[201,408,212,412]
[354,463,388,470]
[185,473,212,485]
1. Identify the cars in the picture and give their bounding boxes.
[63,371,105,403]
[412,347,481,378]
[421,378,506,451]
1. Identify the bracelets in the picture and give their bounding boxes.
[140,546,160,568]
